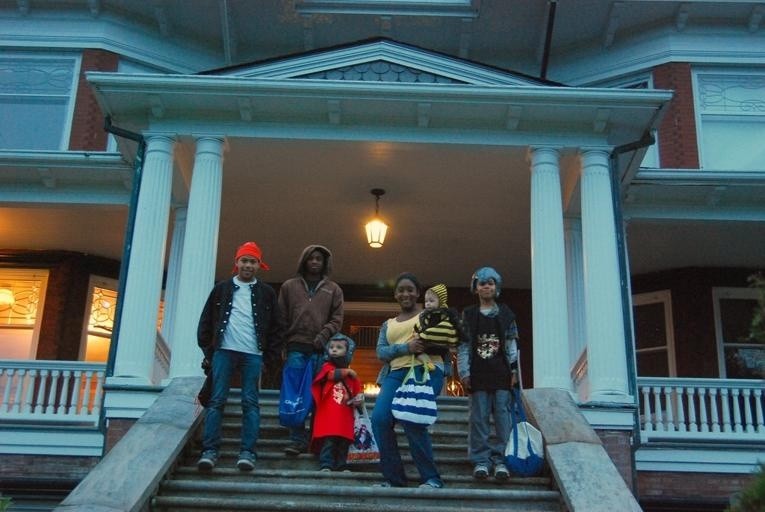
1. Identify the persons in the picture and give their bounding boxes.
[456,267,521,479]
[310,333,364,472]
[278,245,343,454]
[371,272,445,489]
[412,284,460,371]
[196,242,283,471]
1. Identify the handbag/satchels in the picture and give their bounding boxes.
[391,369,438,427]
[278,361,314,427]
[346,418,381,465]
[504,421,544,476]
[198,369,212,407]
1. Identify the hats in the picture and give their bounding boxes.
[323,333,355,367]
[430,283,448,308]
[471,267,501,297]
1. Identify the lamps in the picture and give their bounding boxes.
[363,186,389,250]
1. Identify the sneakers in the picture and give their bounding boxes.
[372,481,391,487]
[473,465,489,478]
[197,449,218,468]
[237,451,255,470]
[321,467,352,472]
[420,484,433,489]
[283,445,308,454]
[494,464,510,479]
